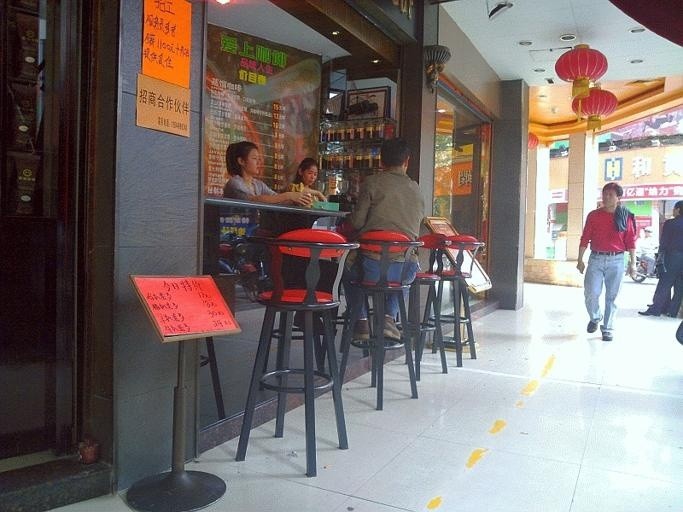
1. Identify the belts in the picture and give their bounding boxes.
[591,251,625,255]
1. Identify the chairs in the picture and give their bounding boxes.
[234,229,360,479]
[430,235,486,366]
[402,233,451,380]
[339,230,425,411]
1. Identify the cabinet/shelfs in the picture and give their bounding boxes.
[319,135,385,202]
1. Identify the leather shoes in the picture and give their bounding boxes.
[639,305,677,317]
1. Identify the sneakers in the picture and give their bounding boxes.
[294,311,337,335]
[383,315,401,340]
[352,320,370,340]
[587,314,603,333]
[602,332,612,340]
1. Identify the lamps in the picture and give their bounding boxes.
[425,42,452,92]
[486,0,514,21]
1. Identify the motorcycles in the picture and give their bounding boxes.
[216,235,260,303]
[629,245,659,283]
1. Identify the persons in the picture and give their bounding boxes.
[288,156,328,207]
[638,200,682,318]
[334,137,428,342]
[576,181,638,343]
[222,141,313,206]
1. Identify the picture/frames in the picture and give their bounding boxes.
[339,86,391,119]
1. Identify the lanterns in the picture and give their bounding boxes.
[555,45,608,121]
[529,130,539,151]
[572,82,618,145]
